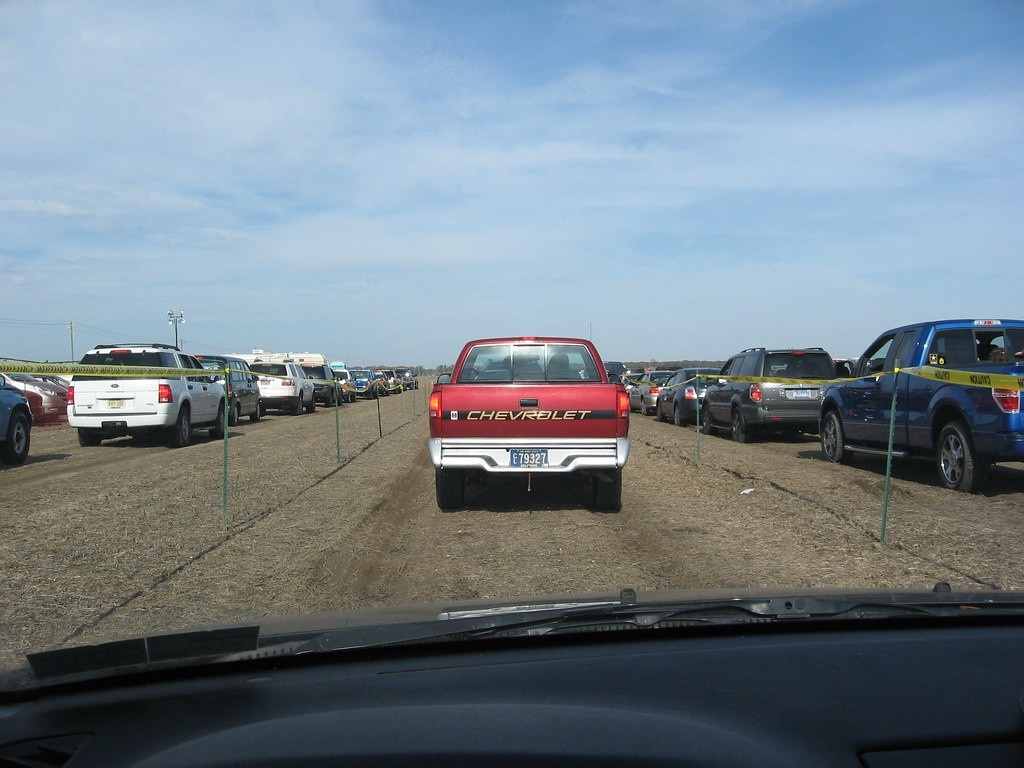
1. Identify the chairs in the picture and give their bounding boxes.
[265,368,270,373]
[277,368,284,374]
[976,343,1008,362]
[481,353,580,380]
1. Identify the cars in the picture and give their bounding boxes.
[0,373,71,469]
[602,358,871,427]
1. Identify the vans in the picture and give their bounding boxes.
[195,354,261,426]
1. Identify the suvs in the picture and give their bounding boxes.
[819,320,1024,492]
[300,363,355,407]
[250,359,317,415]
[348,369,419,399]
[66,343,226,449]
[700,348,838,445]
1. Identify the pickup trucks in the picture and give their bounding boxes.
[428,337,631,512]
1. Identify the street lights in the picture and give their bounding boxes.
[167,309,185,346]
